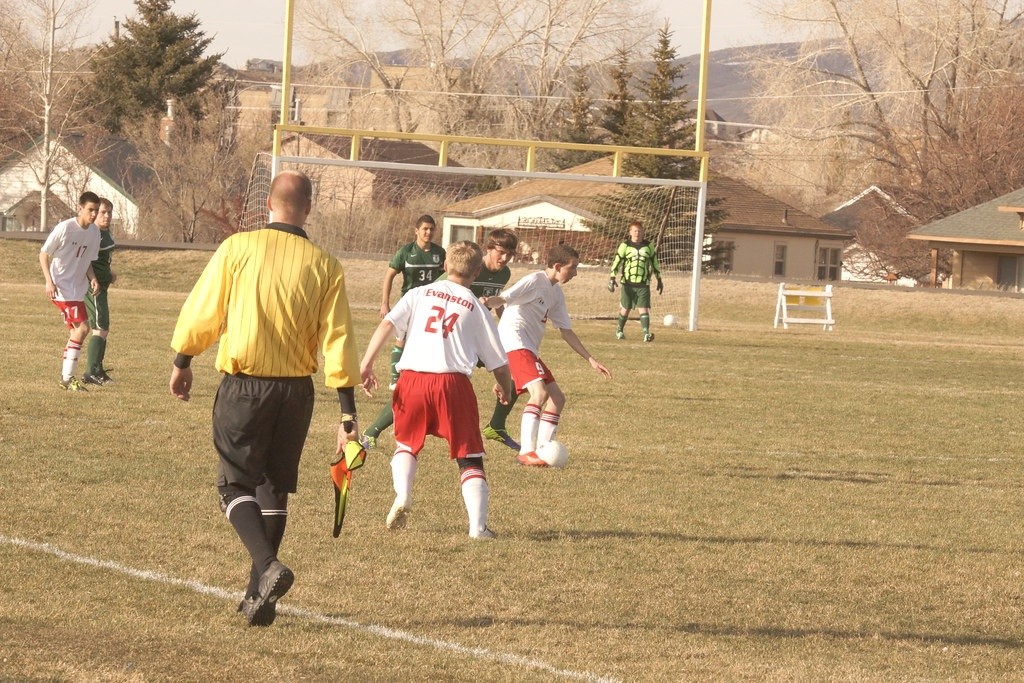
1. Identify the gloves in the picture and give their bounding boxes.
[608,279,619,292]
[657,280,664,294]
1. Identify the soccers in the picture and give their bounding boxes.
[537,440,570,466]
[663,314,675,326]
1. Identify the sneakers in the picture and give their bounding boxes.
[79,372,107,387]
[483,424,520,450]
[59,375,88,393]
[515,451,548,468]
[249,562,294,628]
[94,372,115,384]
[644,333,654,343]
[236,596,260,617]
[359,429,377,449]
[616,331,625,340]
[388,494,412,529]
[468,526,494,540]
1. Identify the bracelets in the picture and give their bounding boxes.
[340,415,358,423]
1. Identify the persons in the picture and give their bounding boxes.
[169,168,359,628]
[360,239,512,539]
[39,191,100,393]
[380,214,447,391]
[81,198,117,386]
[360,228,521,455]
[478,244,613,468]
[608,221,664,342]
